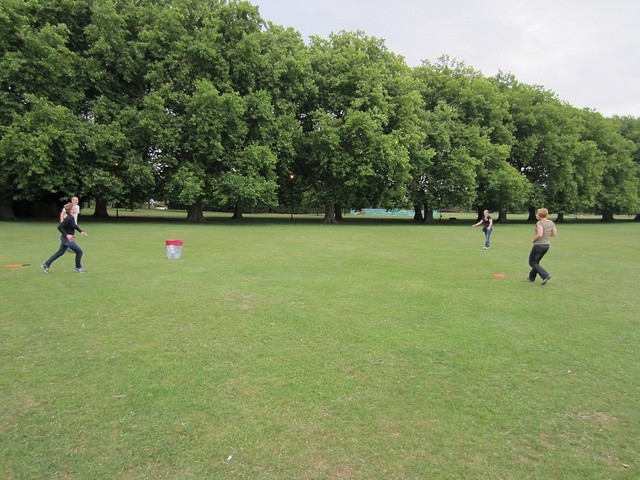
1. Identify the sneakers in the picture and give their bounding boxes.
[74,267,87,273]
[541,275,551,284]
[40,263,49,274]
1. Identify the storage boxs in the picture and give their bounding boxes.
[165,240,183,259]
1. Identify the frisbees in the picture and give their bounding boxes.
[492,273,505,277]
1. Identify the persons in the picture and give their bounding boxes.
[60,193,80,222]
[39,204,82,275]
[527,207,557,287]
[472,209,494,250]
